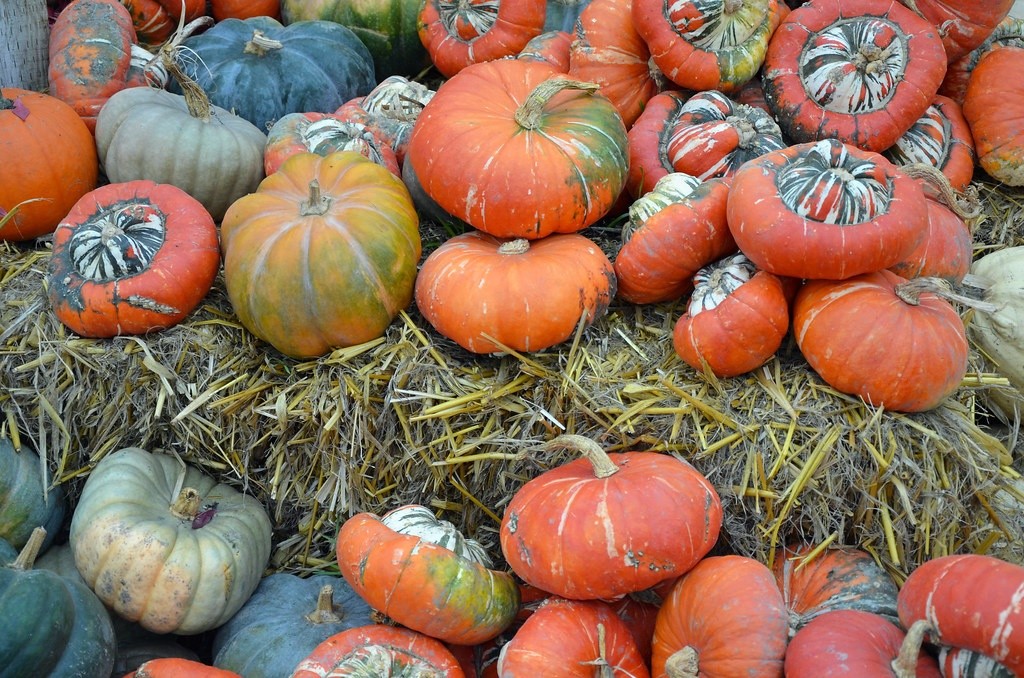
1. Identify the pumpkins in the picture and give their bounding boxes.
[0,0,1024,678]
